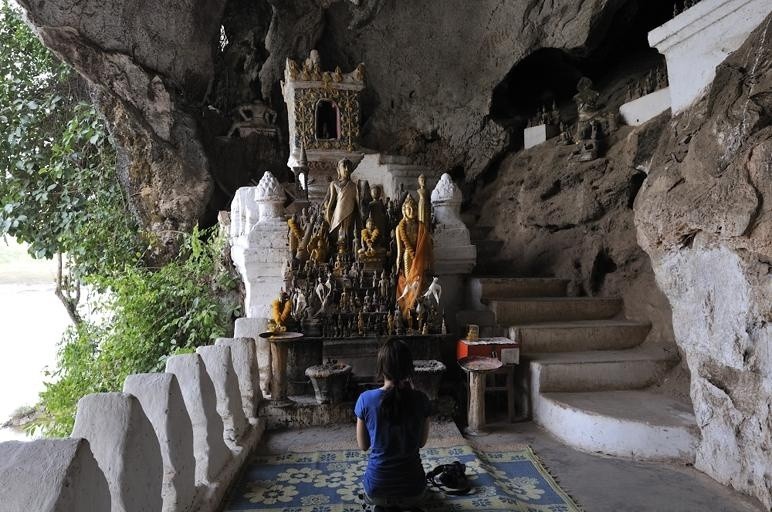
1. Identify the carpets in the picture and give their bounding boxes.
[221,443,586,512]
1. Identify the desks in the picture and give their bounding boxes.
[457,355,503,437]
[259,332,304,410]
[457,337,521,423]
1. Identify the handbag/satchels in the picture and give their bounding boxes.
[439,460,468,489]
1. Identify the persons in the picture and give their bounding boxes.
[354,336,435,512]
[269,154,448,335]
